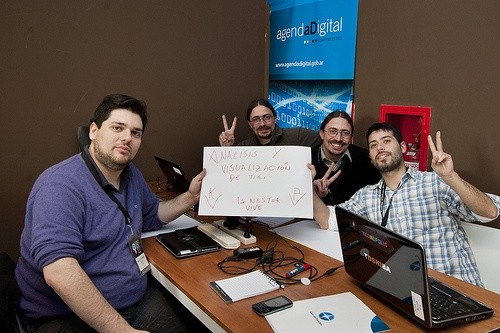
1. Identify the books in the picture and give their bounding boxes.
[156,227,222,258]
[209,269,281,304]
[265,292,391,333]
[367,246,424,306]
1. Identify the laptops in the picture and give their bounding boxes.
[153,156,190,191]
[334,205,493,331]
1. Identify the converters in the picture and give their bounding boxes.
[233,246,263,260]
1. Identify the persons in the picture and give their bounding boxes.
[307,122,500,288]
[15,94,206,333]
[312,111,383,205]
[219,98,323,147]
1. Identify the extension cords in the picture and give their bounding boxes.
[214,220,258,244]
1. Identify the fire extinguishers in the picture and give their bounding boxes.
[403,143,419,171]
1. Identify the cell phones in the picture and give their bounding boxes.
[251,296,293,317]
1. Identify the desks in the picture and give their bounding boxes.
[138,180,500,333]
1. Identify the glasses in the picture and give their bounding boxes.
[323,129,352,138]
[249,115,276,123]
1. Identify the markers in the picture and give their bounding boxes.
[286,263,310,278]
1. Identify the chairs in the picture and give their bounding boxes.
[459,219,500,296]
[0,251,25,333]
